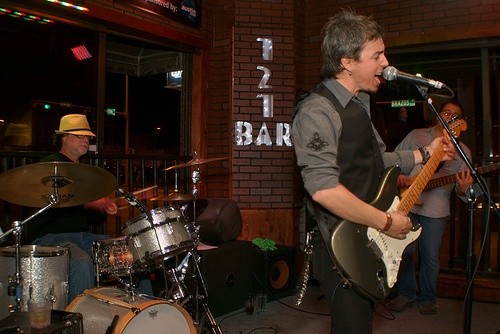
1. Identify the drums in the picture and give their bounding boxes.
[0,244,69,317]
[64,286,197,334]
[120,204,194,270]
[92,236,152,277]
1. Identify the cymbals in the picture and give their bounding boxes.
[0,161,118,208]
[149,192,194,201]
[161,157,232,171]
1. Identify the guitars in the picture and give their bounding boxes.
[322,114,468,303]
[397,161,500,208]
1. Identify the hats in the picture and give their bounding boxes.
[55,113,96,136]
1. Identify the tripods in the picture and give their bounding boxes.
[181,164,223,334]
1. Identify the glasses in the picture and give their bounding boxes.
[441,110,459,119]
[66,133,93,139]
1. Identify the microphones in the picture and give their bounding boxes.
[382,66,443,90]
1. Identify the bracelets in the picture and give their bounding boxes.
[457,189,466,196]
[419,146,430,164]
[377,212,393,231]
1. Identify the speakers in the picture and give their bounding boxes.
[254,244,298,301]
[190,240,256,326]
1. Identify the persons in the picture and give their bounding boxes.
[291,10,454,334]
[390,100,477,315]
[34,115,153,305]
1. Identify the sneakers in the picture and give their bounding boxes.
[388,296,416,312]
[420,302,437,315]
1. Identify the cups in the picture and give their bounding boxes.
[27,299,51,329]
[244,292,267,314]
